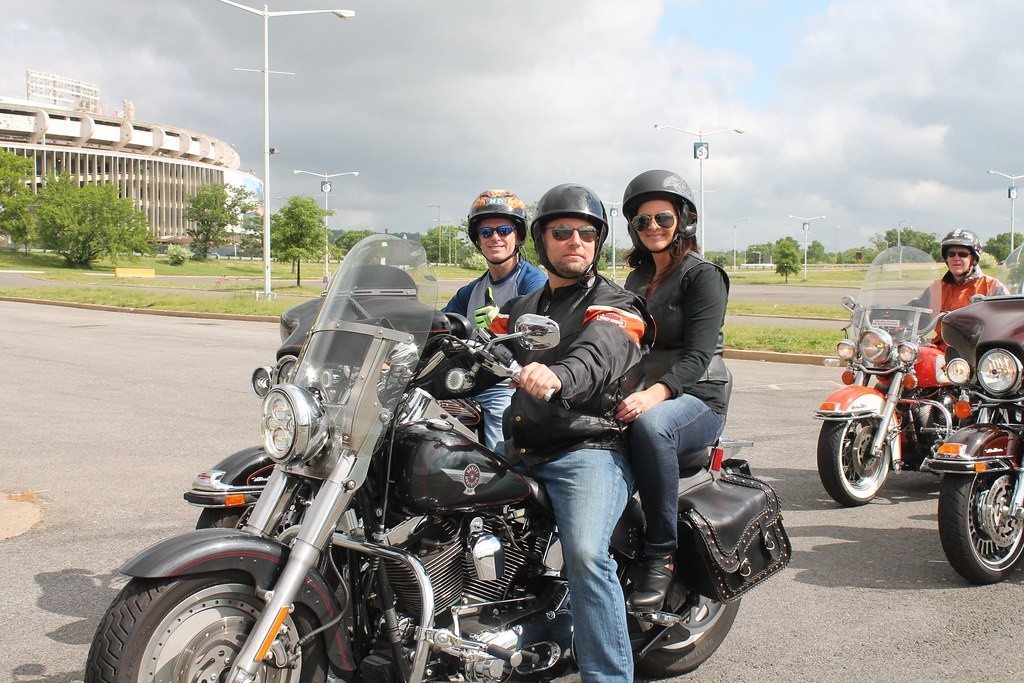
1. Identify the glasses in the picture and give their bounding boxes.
[945,251,973,258]
[631,212,679,232]
[542,224,599,243]
[476,225,518,238]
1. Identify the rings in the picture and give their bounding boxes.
[635,408,642,414]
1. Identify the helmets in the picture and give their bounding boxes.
[467,190,528,246]
[530,183,609,244]
[940,229,981,262]
[622,169,697,224]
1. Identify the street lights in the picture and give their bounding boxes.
[427,205,441,264]
[654,123,744,261]
[292,169,359,283]
[734,219,748,269]
[432,220,451,263]
[898,218,911,281]
[218,0,356,295]
[786,214,827,279]
[987,170,1024,254]
[442,232,457,263]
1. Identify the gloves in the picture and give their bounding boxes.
[474,287,499,330]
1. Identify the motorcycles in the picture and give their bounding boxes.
[811,246,960,507]
[929,244,1024,586]
[85,234,794,683]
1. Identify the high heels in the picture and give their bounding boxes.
[626,554,677,614]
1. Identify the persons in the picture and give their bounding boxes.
[606,170,733,611]
[431,189,553,469]
[403,182,647,683]
[898,228,1011,324]
